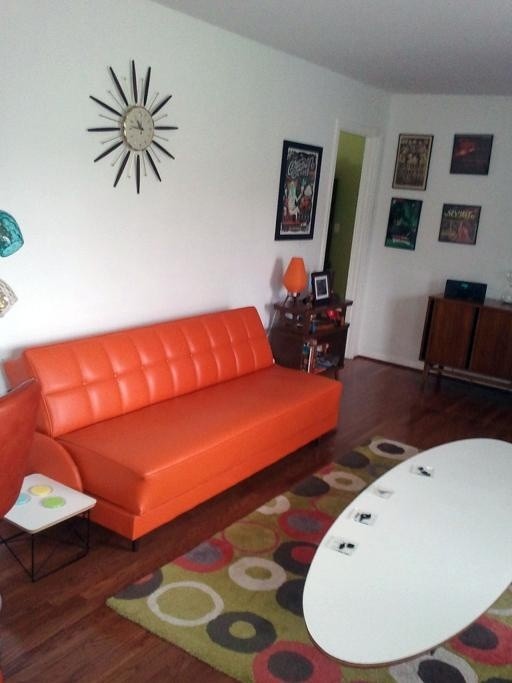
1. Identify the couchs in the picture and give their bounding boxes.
[4,305,343,551]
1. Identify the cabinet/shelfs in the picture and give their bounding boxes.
[271,297,353,380]
[417,293,512,385]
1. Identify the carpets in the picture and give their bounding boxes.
[105,434,512,683]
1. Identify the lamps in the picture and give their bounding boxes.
[282,257,308,308]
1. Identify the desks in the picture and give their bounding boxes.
[0,472,97,582]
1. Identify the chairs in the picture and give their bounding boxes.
[0,376,42,519]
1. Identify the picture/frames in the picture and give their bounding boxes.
[384,197,423,250]
[391,133,434,190]
[449,134,494,175]
[311,271,331,304]
[438,203,481,245]
[274,139,323,241]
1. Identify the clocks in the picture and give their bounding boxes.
[121,106,155,152]
